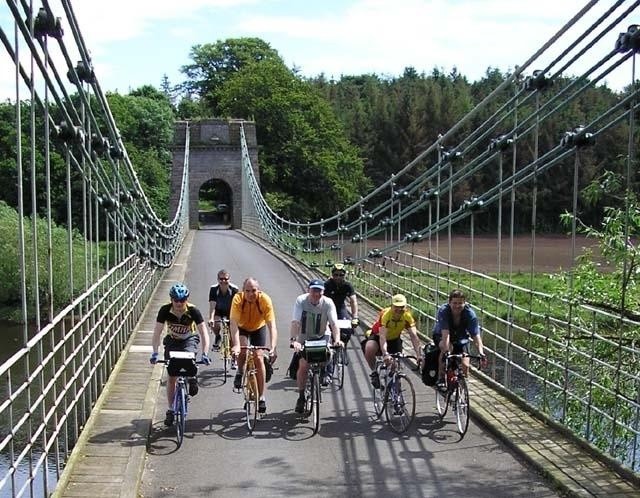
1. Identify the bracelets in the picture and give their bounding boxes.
[290,337,297,341]
[382,352,388,357]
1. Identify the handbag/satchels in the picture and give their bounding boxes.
[421,342,441,387]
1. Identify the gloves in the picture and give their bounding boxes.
[351,318,360,328]
[148,352,161,366]
[200,353,213,367]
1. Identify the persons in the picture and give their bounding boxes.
[229,277,277,413]
[432,290,486,386]
[289,279,345,413]
[324,263,359,384]
[149,284,212,425]
[360,294,426,415]
[208,270,239,370]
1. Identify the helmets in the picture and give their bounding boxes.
[170,284,191,299]
[331,263,347,275]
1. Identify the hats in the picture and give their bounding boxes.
[307,278,326,292]
[390,294,407,309]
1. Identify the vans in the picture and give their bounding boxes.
[215,203,228,213]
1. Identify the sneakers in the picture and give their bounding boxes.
[211,333,222,352]
[369,370,382,389]
[294,397,308,414]
[187,376,200,398]
[234,370,244,390]
[257,397,266,414]
[163,409,176,427]
